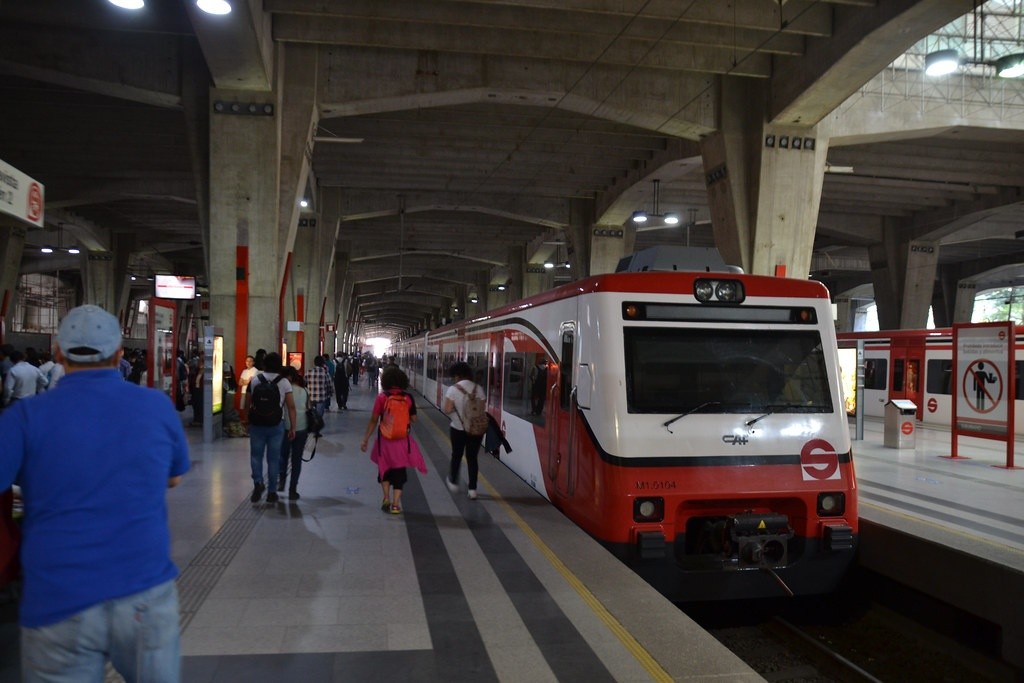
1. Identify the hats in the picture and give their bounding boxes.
[134,348,142,355]
[58,304,122,364]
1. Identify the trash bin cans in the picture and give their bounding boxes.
[882,398,918,450]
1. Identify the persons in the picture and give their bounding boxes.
[529,358,549,416]
[304,351,347,416]
[361,368,417,514]
[348,351,400,386]
[0,343,147,404]
[445,362,487,499]
[0,305,190,683]
[222,349,268,410]
[176,350,203,425]
[242,353,311,503]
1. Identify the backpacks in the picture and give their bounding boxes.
[378,391,412,440]
[452,382,489,435]
[333,357,347,381]
[223,360,232,379]
[177,359,187,380]
[248,373,287,427]
[352,357,359,368]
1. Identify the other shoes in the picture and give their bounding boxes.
[338,401,347,409]
[266,491,278,502]
[189,420,202,427]
[446,476,459,492]
[250,481,266,503]
[467,489,477,499]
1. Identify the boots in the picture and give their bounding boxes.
[289,474,300,500]
[276,473,288,491]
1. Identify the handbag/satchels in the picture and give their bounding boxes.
[306,407,325,432]
[225,421,250,437]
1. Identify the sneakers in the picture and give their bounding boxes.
[391,503,402,513]
[381,495,390,511]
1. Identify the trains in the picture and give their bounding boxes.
[388,270,859,599]
